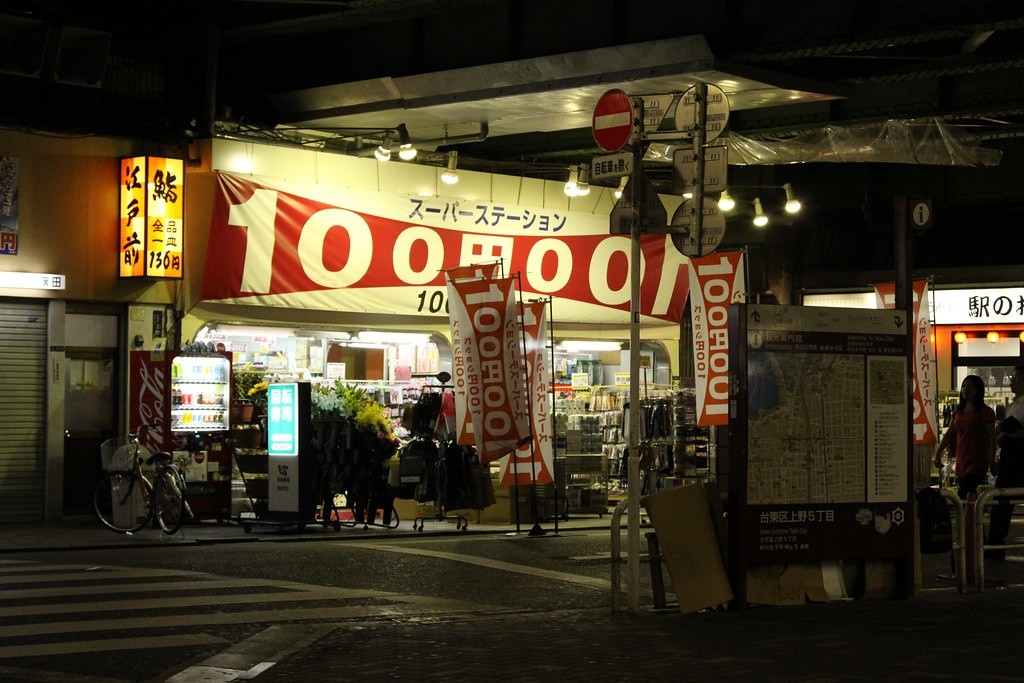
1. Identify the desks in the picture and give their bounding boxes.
[566,454,608,517]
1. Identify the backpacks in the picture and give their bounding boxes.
[917,487,956,575]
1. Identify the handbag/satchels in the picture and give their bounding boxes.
[945,428,957,458]
[386,388,442,490]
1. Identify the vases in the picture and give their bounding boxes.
[258,415,268,429]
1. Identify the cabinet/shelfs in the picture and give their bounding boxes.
[575,385,710,500]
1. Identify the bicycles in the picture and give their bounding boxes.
[92,424,187,542]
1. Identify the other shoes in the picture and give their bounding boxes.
[984,552,1007,559]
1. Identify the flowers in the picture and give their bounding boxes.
[248,382,270,415]
[310,376,404,468]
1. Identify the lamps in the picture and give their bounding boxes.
[615,177,628,198]
[783,183,801,212]
[718,187,735,210]
[564,164,590,197]
[442,151,459,184]
[375,123,417,160]
[752,198,768,226]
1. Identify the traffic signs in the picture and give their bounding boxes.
[591,152,634,179]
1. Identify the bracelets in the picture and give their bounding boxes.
[934,458,941,463]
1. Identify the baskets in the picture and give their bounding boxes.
[101,436,138,471]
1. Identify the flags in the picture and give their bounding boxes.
[873,279,937,448]
[442,262,557,488]
[688,249,748,427]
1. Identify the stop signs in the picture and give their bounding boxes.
[592,89,633,152]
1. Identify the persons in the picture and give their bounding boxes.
[982,366,1024,559]
[934,375,999,544]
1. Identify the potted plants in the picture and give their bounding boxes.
[232,368,253,422]
[232,426,261,446]
[246,474,268,496]
[240,449,268,470]
